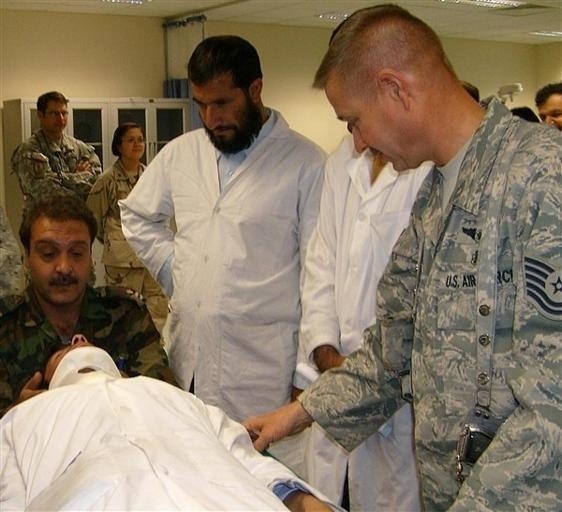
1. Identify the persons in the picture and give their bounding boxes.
[0,195,183,419]
[535,83,562,131]
[116,36,329,484]
[460,79,480,104]
[10,92,102,289]
[511,108,540,124]
[85,123,170,336]
[241,5,562,512]
[0,334,334,512]
[300,135,435,512]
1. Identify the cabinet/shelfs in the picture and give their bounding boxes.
[1,97,111,291]
[110,98,192,170]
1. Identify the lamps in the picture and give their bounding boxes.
[498,82,522,109]
